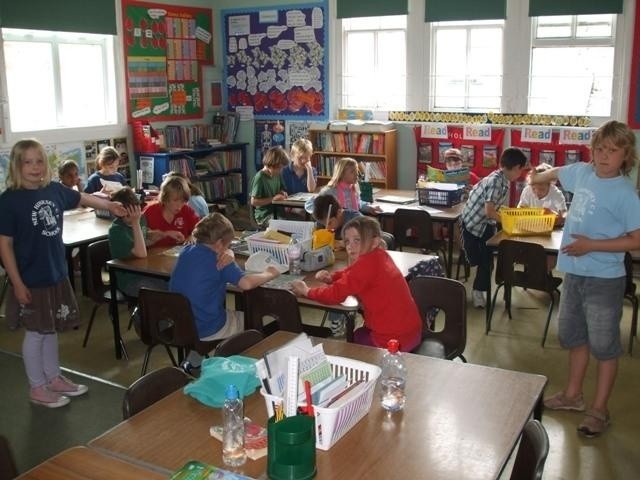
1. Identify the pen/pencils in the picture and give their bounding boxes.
[272,400,285,422]
[136,170,143,189]
[304,381,317,416]
[326,204,332,228]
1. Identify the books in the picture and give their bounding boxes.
[311,153,341,176]
[141,114,237,152]
[228,268,305,290]
[355,160,387,181]
[209,417,268,460]
[163,148,246,179]
[318,133,386,154]
[157,245,185,259]
[427,165,470,194]
[285,191,318,203]
[190,180,214,203]
[308,120,394,132]
[210,172,243,202]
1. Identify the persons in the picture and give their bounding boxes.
[304,157,395,251]
[312,195,364,250]
[106,187,184,300]
[249,146,290,227]
[517,162,570,278]
[168,213,282,379]
[0,138,128,407]
[290,216,423,353]
[527,121,640,438]
[281,137,317,217]
[56,159,85,192]
[142,175,203,246]
[83,146,129,197]
[161,171,208,218]
[459,146,527,310]
[427,148,482,251]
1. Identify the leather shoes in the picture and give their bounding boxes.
[29,391,71,409]
[47,382,88,397]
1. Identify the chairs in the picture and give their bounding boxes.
[122,367,189,422]
[83,239,139,359]
[622,252,638,359]
[485,239,564,347]
[392,208,449,276]
[508,420,550,480]
[138,287,225,377]
[214,330,264,358]
[408,275,469,363]
[243,286,303,333]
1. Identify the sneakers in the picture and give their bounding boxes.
[471,288,486,309]
[543,390,586,412]
[576,409,612,439]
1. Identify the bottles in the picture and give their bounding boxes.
[287,234,301,275]
[380,340,407,412]
[416,175,424,201]
[222,385,247,467]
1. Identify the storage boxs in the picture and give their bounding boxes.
[416,181,464,207]
[93,193,142,219]
[259,354,382,451]
[498,207,558,236]
[243,229,314,270]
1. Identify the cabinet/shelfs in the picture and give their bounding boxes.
[307,126,399,192]
[134,141,250,204]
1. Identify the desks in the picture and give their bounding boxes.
[485,229,640,264]
[88,330,548,480]
[61,191,228,296]
[271,186,467,277]
[106,229,440,360]
[16,444,171,480]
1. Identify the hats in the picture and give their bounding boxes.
[442,148,465,163]
[110,188,142,219]
[244,250,292,277]
